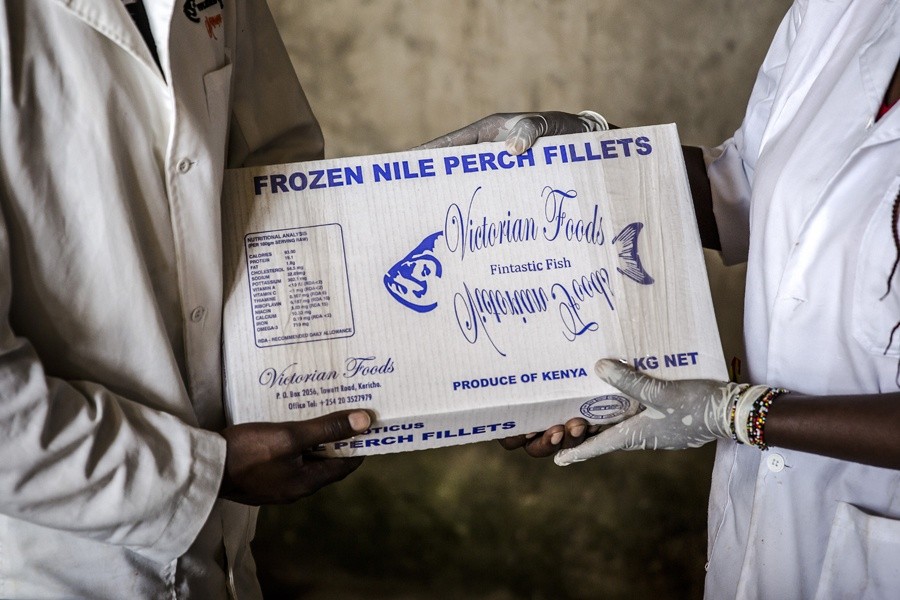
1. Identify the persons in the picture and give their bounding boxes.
[416,0,900,600]
[0,0,376,600]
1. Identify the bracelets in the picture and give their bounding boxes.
[731,385,790,451]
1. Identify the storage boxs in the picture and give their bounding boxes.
[218,122,729,457]
